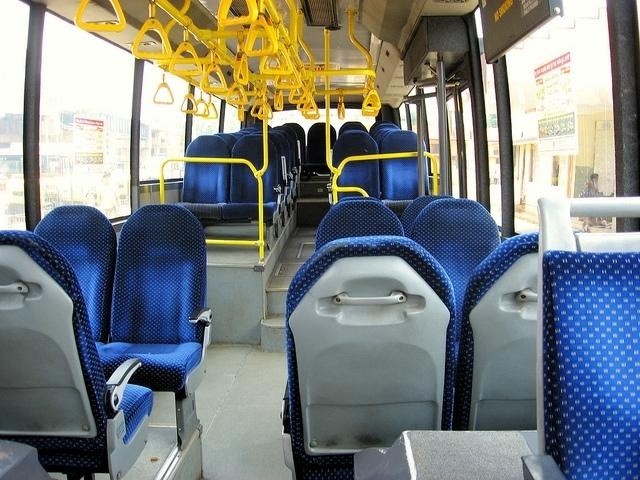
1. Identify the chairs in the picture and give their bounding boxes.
[326,121,431,219]
[175,122,301,251]
[283,122,336,181]
[0,203,214,480]
[280,195,639,480]
